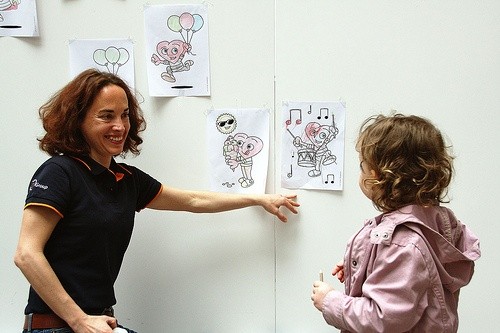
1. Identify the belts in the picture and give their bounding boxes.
[24,306,114,330]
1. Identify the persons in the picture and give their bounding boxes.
[311,115,481,333]
[14,69,300,333]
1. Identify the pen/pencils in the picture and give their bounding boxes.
[320,267,323,282]
